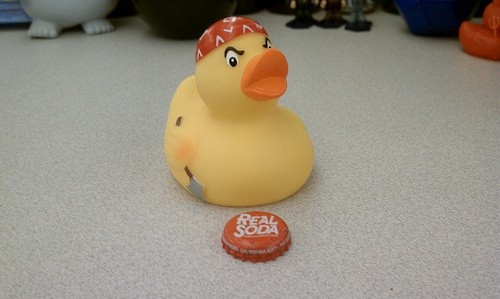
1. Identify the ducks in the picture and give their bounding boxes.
[163,16,316,207]
